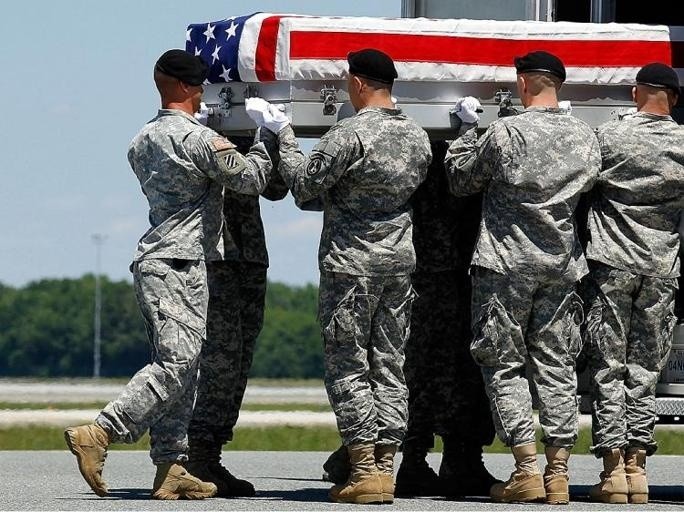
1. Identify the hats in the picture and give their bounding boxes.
[635,63,680,95]
[153,48,212,88]
[514,51,566,82]
[343,50,399,85]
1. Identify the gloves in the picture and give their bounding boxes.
[243,97,267,128]
[195,101,210,127]
[557,101,571,116]
[260,106,289,134]
[454,96,481,124]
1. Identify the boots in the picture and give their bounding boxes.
[588,447,628,503]
[150,462,217,501]
[374,443,396,503]
[186,460,255,497]
[438,450,504,496]
[625,445,649,503]
[543,445,570,505]
[394,450,460,498]
[489,440,546,502]
[328,445,383,504]
[64,421,110,497]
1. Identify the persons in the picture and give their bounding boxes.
[317,130,444,495]
[183,131,288,497]
[581,62,684,504]
[260,48,435,505]
[64,48,272,502]
[443,50,604,507]
[434,130,507,500]
